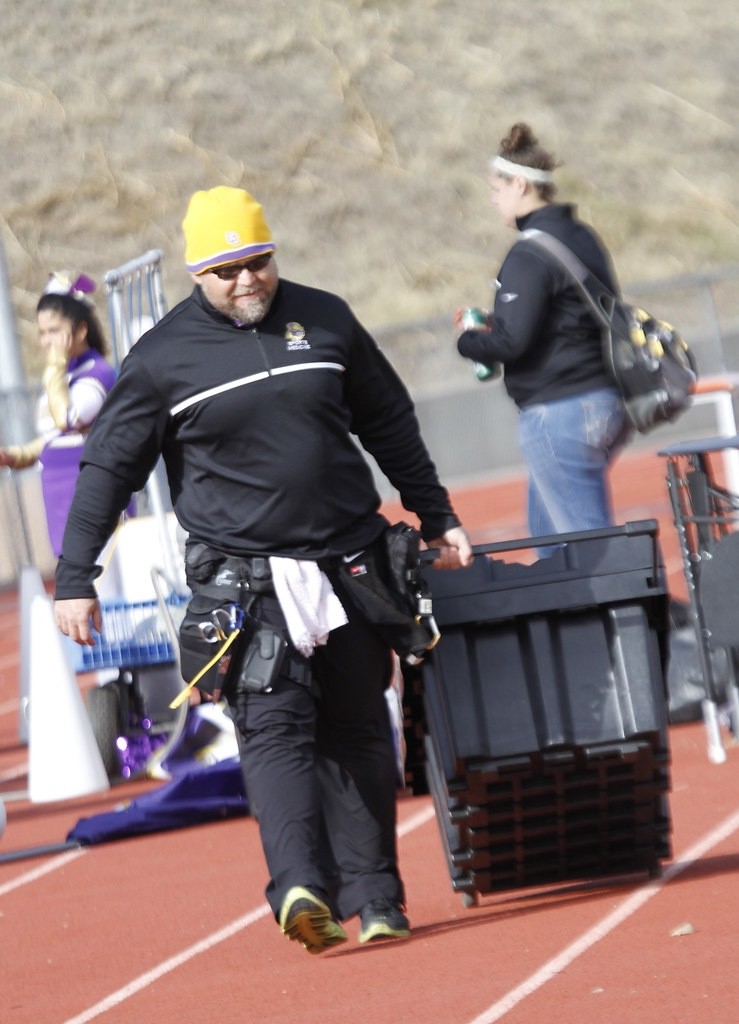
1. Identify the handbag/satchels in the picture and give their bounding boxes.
[603,295,696,432]
[386,521,423,595]
[177,596,247,692]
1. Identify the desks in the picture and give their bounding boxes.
[659,431,739,764]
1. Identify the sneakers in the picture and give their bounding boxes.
[357,899,411,942]
[279,886,348,956]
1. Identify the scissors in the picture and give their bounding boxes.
[198,610,234,643]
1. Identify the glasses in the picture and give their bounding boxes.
[207,253,272,279]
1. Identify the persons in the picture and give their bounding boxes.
[455,123,635,631]
[0,270,140,558]
[53,186,473,955]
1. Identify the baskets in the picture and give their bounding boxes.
[63,594,191,672]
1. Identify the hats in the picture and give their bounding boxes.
[43,267,99,308]
[182,185,276,274]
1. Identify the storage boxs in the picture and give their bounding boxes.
[69,594,183,675]
[398,518,676,906]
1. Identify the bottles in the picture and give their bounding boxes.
[460,306,503,382]
